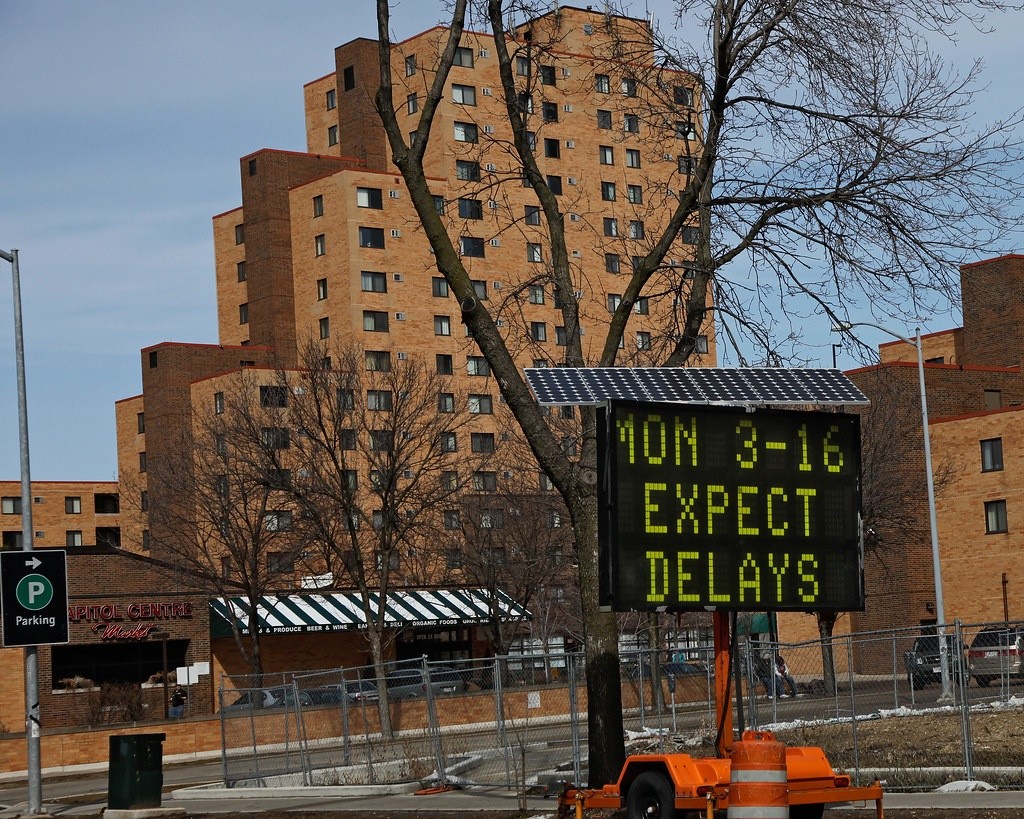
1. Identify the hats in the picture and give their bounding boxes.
[763,653,770,659]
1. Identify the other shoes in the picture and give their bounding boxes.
[791,694,796,700]
[780,694,789,699]
[767,696,773,700]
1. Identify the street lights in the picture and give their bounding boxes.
[827,317,955,704]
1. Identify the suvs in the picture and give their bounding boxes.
[966,623,1024,687]
[903,634,971,691]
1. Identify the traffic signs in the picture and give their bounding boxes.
[0,549,71,648]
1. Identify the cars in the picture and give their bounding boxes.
[215,666,466,716]
[620,659,708,678]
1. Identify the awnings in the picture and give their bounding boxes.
[209,586,537,636]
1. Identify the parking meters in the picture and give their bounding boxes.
[666,671,678,733]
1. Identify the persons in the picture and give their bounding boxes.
[170,684,187,719]
[759,653,804,699]
